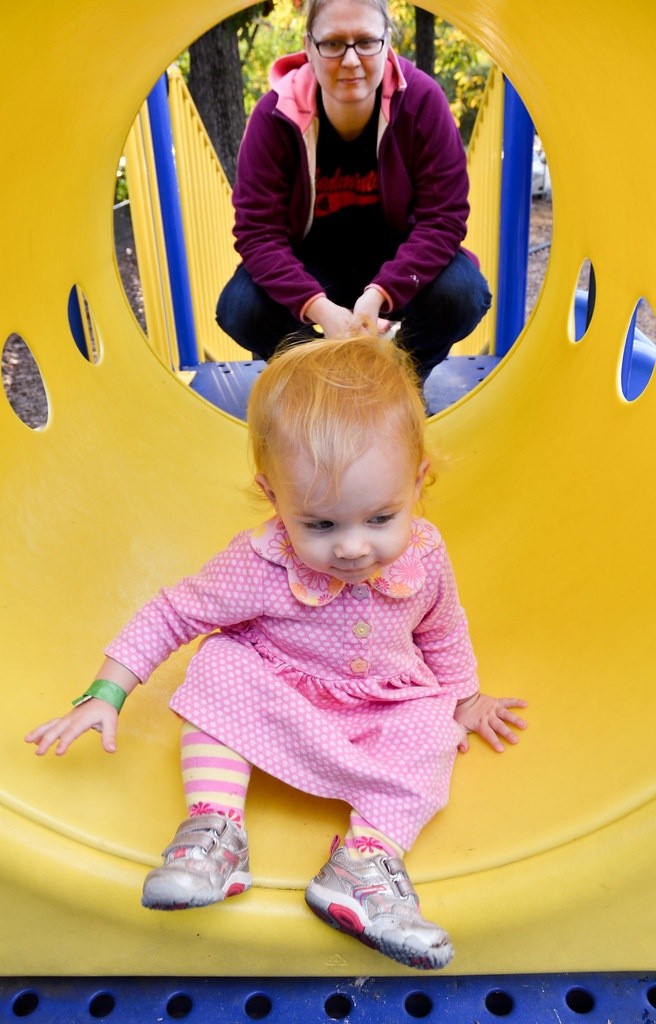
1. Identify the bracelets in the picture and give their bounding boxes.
[70,679,127,714]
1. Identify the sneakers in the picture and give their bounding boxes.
[305,835,454,970]
[141,816,253,911]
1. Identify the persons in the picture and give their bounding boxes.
[24,337,529,970]
[213,1,492,416]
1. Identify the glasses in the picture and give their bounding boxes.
[311,31,386,58]
